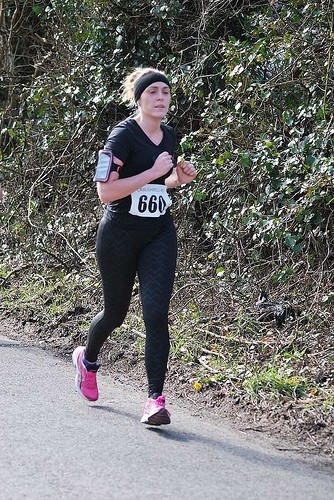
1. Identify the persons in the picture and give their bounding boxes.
[71,68,199,426]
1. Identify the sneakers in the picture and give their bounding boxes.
[71,347,99,402]
[139,392,172,426]
